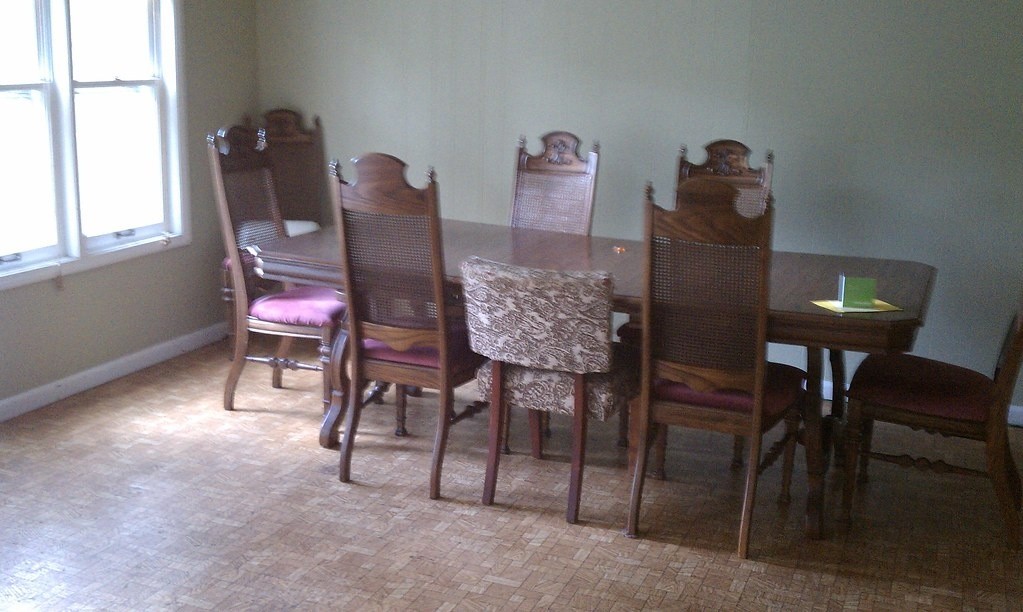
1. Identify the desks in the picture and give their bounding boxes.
[243,215,937,544]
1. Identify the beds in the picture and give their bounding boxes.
[207,107,349,419]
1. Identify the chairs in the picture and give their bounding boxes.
[504,131,604,236]
[326,152,492,501]
[457,254,638,525]
[672,139,777,213]
[622,177,811,562]
[831,299,1023,549]
